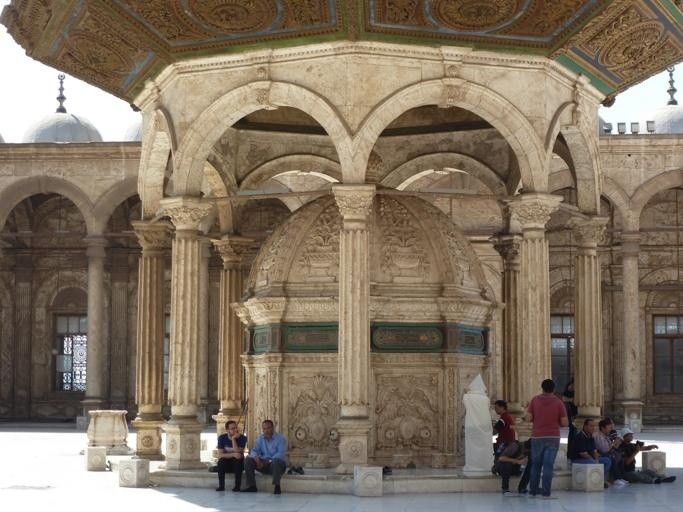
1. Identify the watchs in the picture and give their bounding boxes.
[268,458,272,465]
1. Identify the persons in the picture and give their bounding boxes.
[571,416,677,489]
[215,420,247,491]
[240,419,288,494]
[521,379,569,500]
[562,380,577,459]
[493,438,531,497]
[518,454,530,494]
[493,399,516,443]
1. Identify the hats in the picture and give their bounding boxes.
[621,428,634,438]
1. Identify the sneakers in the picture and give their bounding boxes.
[655,476,676,483]
[215,483,281,494]
[502,490,556,500]
